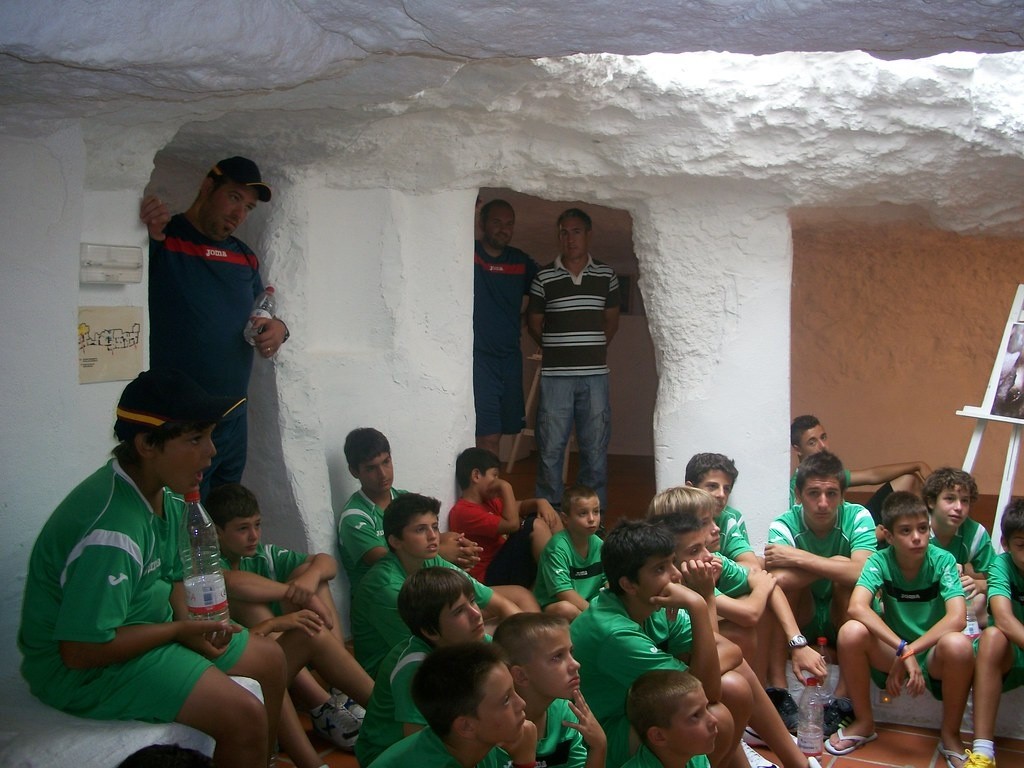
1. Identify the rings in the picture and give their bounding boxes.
[268,347,271,353]
[158,199,161,205]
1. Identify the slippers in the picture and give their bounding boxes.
[824,728,878,754]
[937,742,968,768]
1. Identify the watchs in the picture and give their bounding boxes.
[787,635,808,650]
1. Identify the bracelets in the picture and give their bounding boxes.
[901,650,915,661]
[511,761,537,768]
[275,319,290,344]
[895,640,906,656]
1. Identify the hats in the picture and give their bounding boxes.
[114,369,230,440]
[207,156,271,203]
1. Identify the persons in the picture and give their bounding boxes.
[472,196,542,459]
[11,372,289,768]
[494,611,607,768]
[338,429,829,768]
[365,639,536,768]
[763,414,1024,766]
[526,209,624,538]
[138,155,290,510]
[204,485,376,768]
[618,668,720,768]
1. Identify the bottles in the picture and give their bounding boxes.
[953,574,980,641]
[177,492,230,632]
[243,286,276,346]
[799,677,825,764]
[812,637,832,706]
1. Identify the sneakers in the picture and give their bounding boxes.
[766,687,807,733]
[327,688,367,720]
[741,740,779,768]
[311,701,364,750]
[962,748,997,768]
[823,697,856,739]
